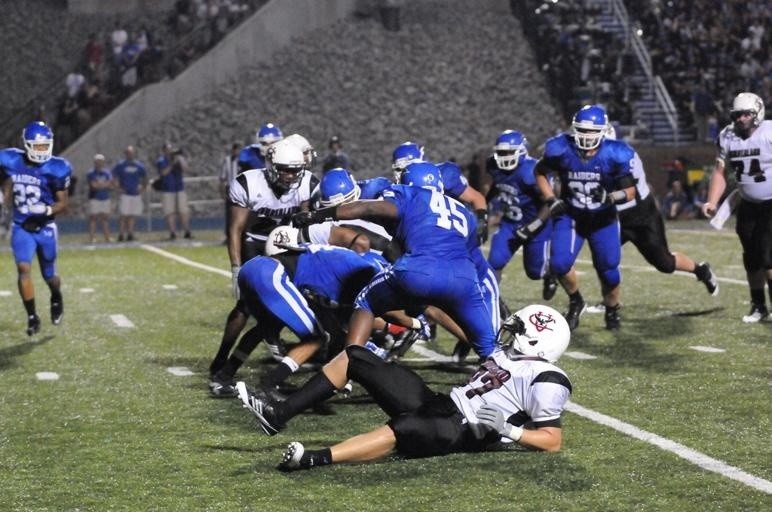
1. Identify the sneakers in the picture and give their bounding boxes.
[602,304,621,330]
[204,372,292,403]
[451,340,470,364]
[697,261,719,297]
[87,230,196,242]
[27,315,41,336]
[742,306,768,323]
[233,379,287,437]
[261,334,289,362]
[564,300,587,330]
[275,441,305,471]
[49,300,65,325]
[541,275,558,301]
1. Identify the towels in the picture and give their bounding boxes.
[709,190,742,233]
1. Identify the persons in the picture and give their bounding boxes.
[1,1,269,201]
[57,142,191,245]
[510,1,771,323]
[234,302,573,471]
[1,121,72,337]
[207,104,720,397]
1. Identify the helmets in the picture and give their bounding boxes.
[570,104,609,133]
[22,121,54,146]
[320,168,361,203]
[392,142,427,168]
[399,162,446,195]
[272,133,313,171]
[264,224,303,259]
[506,303,571,364]
[256,124,284,143]
[729,91,766,127]
[493,128,527,166]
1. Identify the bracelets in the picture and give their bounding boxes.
[506,425,524,444]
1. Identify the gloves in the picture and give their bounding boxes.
[292,204,340,228]
[586,183,614,209]
[472,209,488,246]
[366,313,433,362]
[548,197,569,217]
[476,403,523,443]
[28,201,54,218]
[513,218,545,244]
[231,265,245,302]
